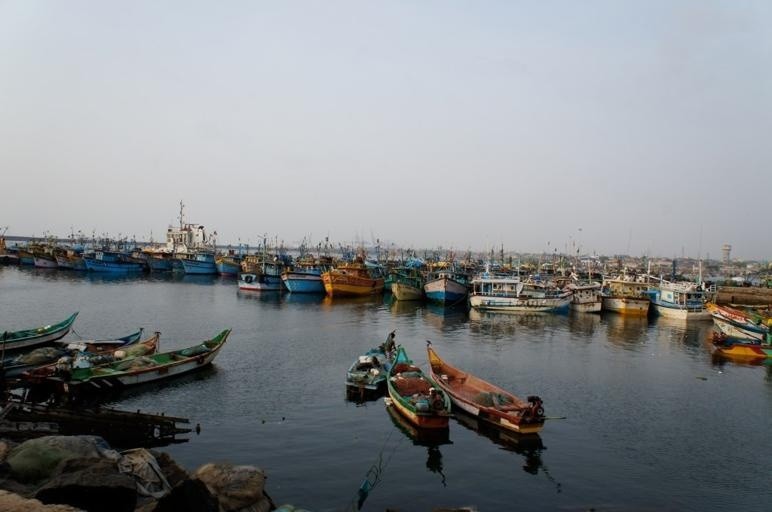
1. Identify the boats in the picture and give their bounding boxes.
[553,259,602,313]
[0,198,574,311]
[600,268,650,318]
[709,353,772,367]
[388,343,453,432]
[425,340,546,436]
[0,327,144,381]
[345,327,399,395]
[345,383,389,404]
[449,399,547,476]
[647,259,713,321]
[20,331,162,393]
[65,359,218,411]
[46,326,233,399]
[704,298,771,347]
[386,405,453,474]
